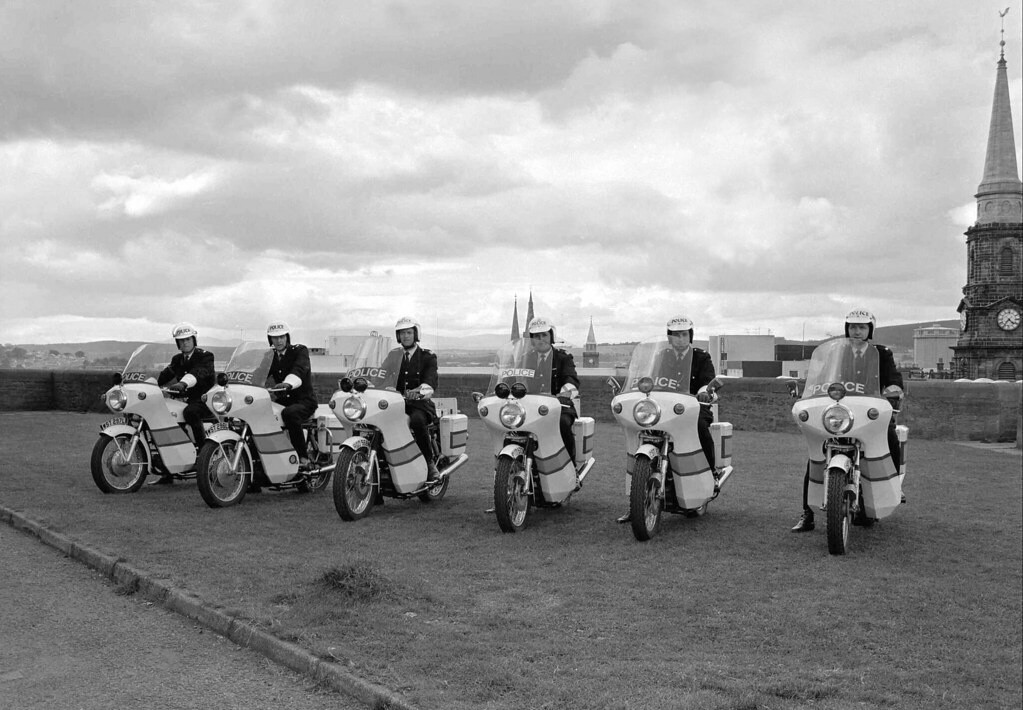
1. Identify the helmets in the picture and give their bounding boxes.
[667,316,693,344]
[528,316,555,345]
[267,321,290,346]
[173,325,198,349]
[845,309,876,340]
[395,316,421,344]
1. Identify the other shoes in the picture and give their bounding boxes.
[427,462,440,481]
[574,478,581,492]
[791,510,814,533]
[616,506,631,522]
[299,457,312,471]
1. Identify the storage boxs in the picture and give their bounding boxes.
[710,422,733,468]
[896,425,909,483]
[440,414,467,457]
[317,415,347,454]
[574,417,596,463]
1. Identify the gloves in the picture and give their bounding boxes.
[170,382,188,397]
[274,383,292,399]
[696,392,711,403]
[556,396,569,405]
[887,397,900,413]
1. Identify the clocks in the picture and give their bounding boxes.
[959,310,967,333]
[997,309,1020,331]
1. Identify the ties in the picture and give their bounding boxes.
[279,354,284,361]
[185,355,188,363]
[539,355,546,370]
[677,353,683,366]
[855,350,862,364]
[405,352,410,366]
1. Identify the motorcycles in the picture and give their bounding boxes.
[607,335,734,541]
[328,336,469,521]
[792,338,910,555]
[472,338,596,533]
[195,340,348,509]
[91,344,218,494]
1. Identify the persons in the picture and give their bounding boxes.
[373,316,440,505]
[792,308,906,532]
[616,315,720,523]
[247,321,318,493]
[147,324,215,484]
[484,316,581,513]
